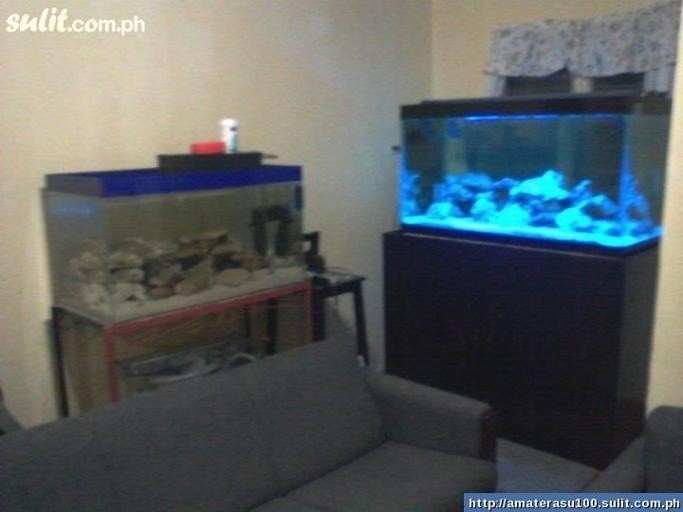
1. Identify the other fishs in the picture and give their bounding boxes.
[485,204,529,227]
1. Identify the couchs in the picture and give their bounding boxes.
[0,340,499,512]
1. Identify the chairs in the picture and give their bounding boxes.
[255,205,368,368]
[587,405,682,492]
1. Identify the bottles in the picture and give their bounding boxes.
[219,117,240,152]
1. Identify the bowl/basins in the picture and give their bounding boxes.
[188,141,226,155]
